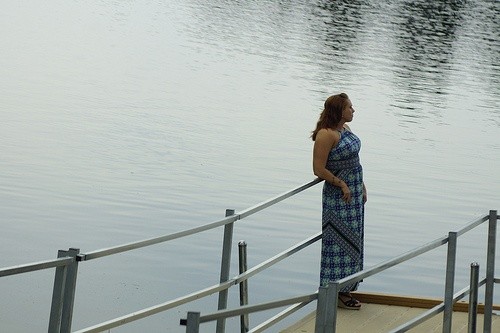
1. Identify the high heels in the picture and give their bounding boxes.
[336,291,361,310]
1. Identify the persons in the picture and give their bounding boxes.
[309,93,368,310]
